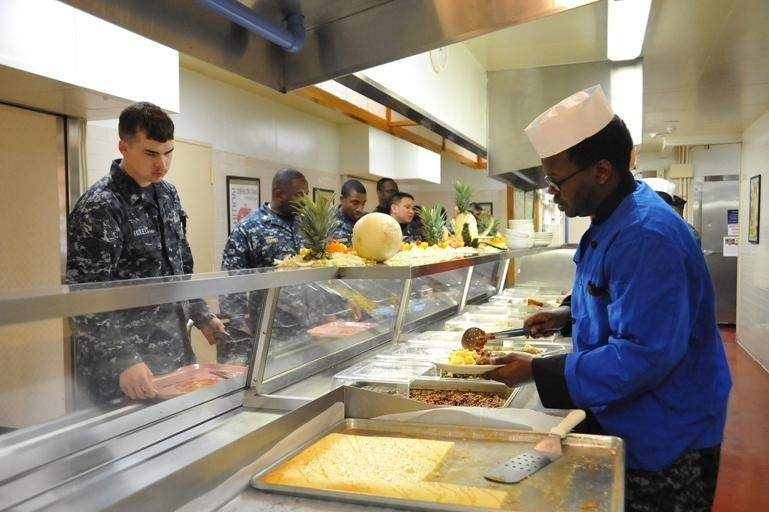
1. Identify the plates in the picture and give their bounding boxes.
[432,349,538,374]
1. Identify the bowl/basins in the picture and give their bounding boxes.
[502,220,553,248]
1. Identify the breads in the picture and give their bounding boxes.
[257,430,508,511]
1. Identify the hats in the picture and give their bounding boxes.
[524,85,615,159]
[639,177,676,201]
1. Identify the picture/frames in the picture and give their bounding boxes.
[474,203,493,216]
[749,175,760,244]
[313,187,335,207]
[226,176,260,237]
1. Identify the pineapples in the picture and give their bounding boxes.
[411,201,450,248]
[451,178,479,246]
[288,185,345,263]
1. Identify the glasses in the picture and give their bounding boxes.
[544,157,605,191]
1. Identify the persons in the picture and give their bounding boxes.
[65,102,230,405]
[375,178,485,244]
[479,114,733,512]
[213,170,361,365]
[670,195,702,250]
[325,178,373,251]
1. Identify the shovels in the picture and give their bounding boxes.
[485,409,586,483]
